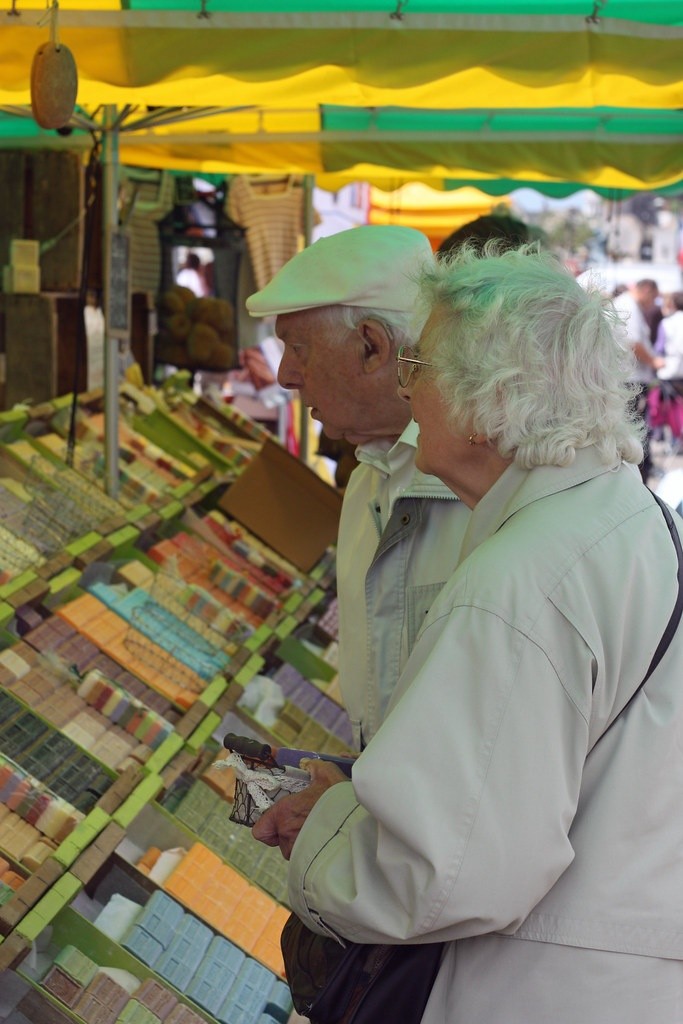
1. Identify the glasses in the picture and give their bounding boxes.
[396,345,437,388]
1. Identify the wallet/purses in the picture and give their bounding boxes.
[228,762,312,828]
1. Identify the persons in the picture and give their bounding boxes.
[247,215,683,1024]
[175,250,209,302]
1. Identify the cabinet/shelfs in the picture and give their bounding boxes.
[1,376,357,1024]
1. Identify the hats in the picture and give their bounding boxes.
[246,225,435,317]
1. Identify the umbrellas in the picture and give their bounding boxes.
[0,0,683,498]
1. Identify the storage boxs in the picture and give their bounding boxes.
[217,432,346,573]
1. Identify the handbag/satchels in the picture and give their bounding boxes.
[280,911,447,1024]
